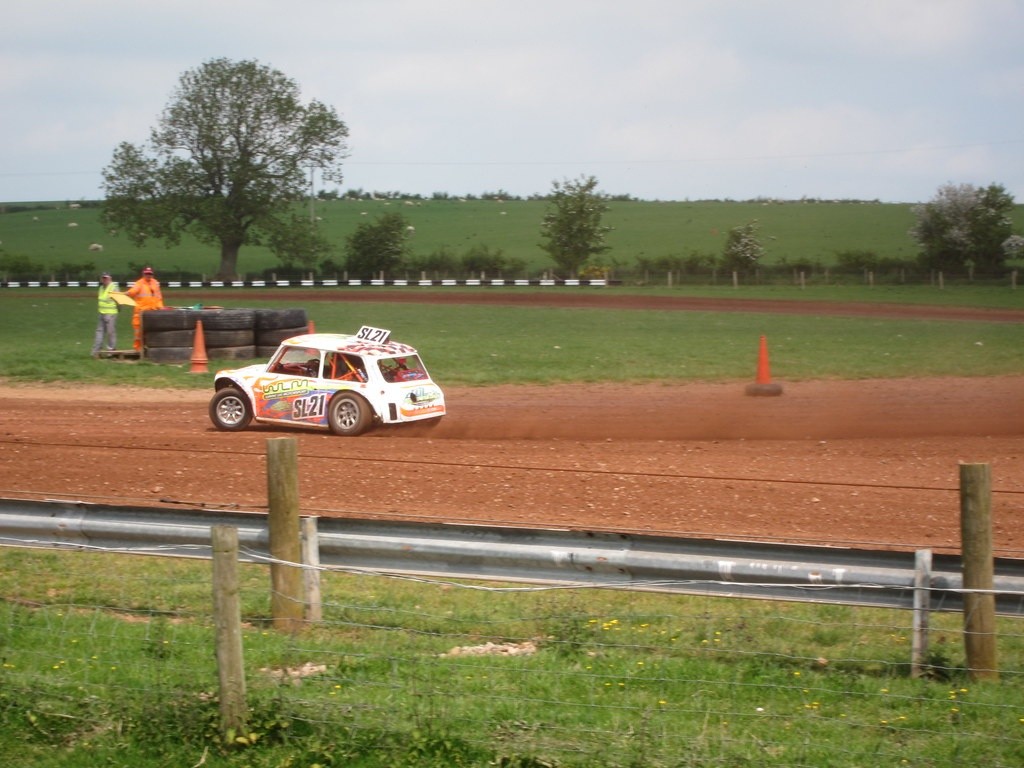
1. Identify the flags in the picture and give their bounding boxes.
[108,293,136,306]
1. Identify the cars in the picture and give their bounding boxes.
[208,326,447,436]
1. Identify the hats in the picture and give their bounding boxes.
[143,267,153,275]
[101,271,111,278]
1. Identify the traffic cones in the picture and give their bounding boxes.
[307,320,316,334]
[745,336,782,397]
[189,320,209,373]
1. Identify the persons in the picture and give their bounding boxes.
[125,267,164,350]
[304,352,346,379]
[91,273,120,356]
